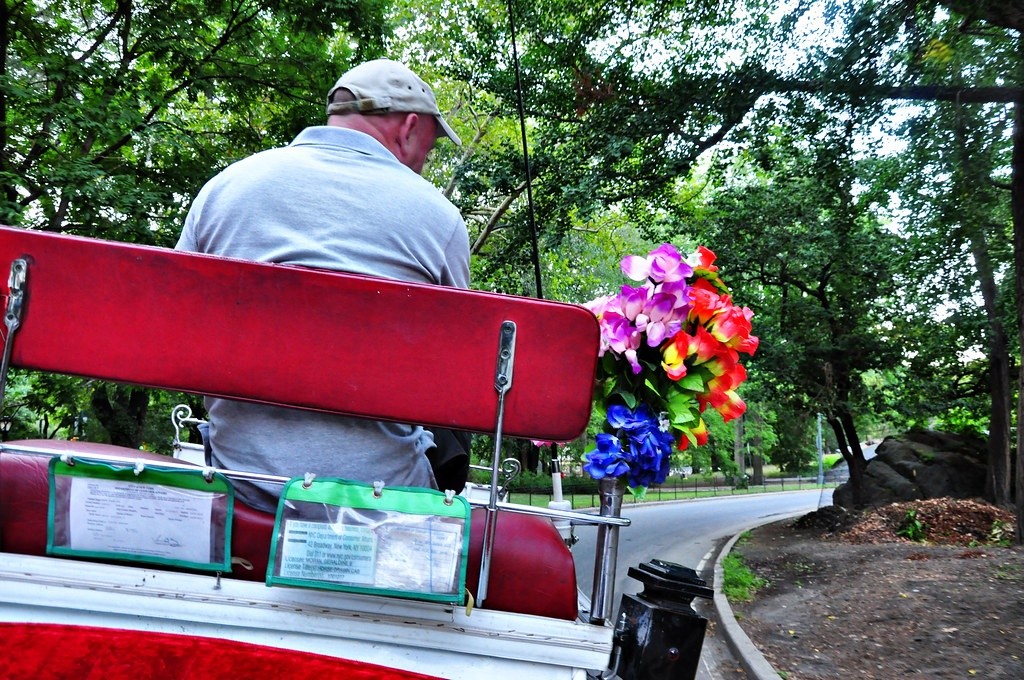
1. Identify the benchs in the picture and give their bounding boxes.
[0,226,632,671]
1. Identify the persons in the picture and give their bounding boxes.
[172,55,472,526]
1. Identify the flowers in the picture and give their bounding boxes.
[583,242,760,499]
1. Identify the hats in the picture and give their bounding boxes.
[325,59,463,147]
